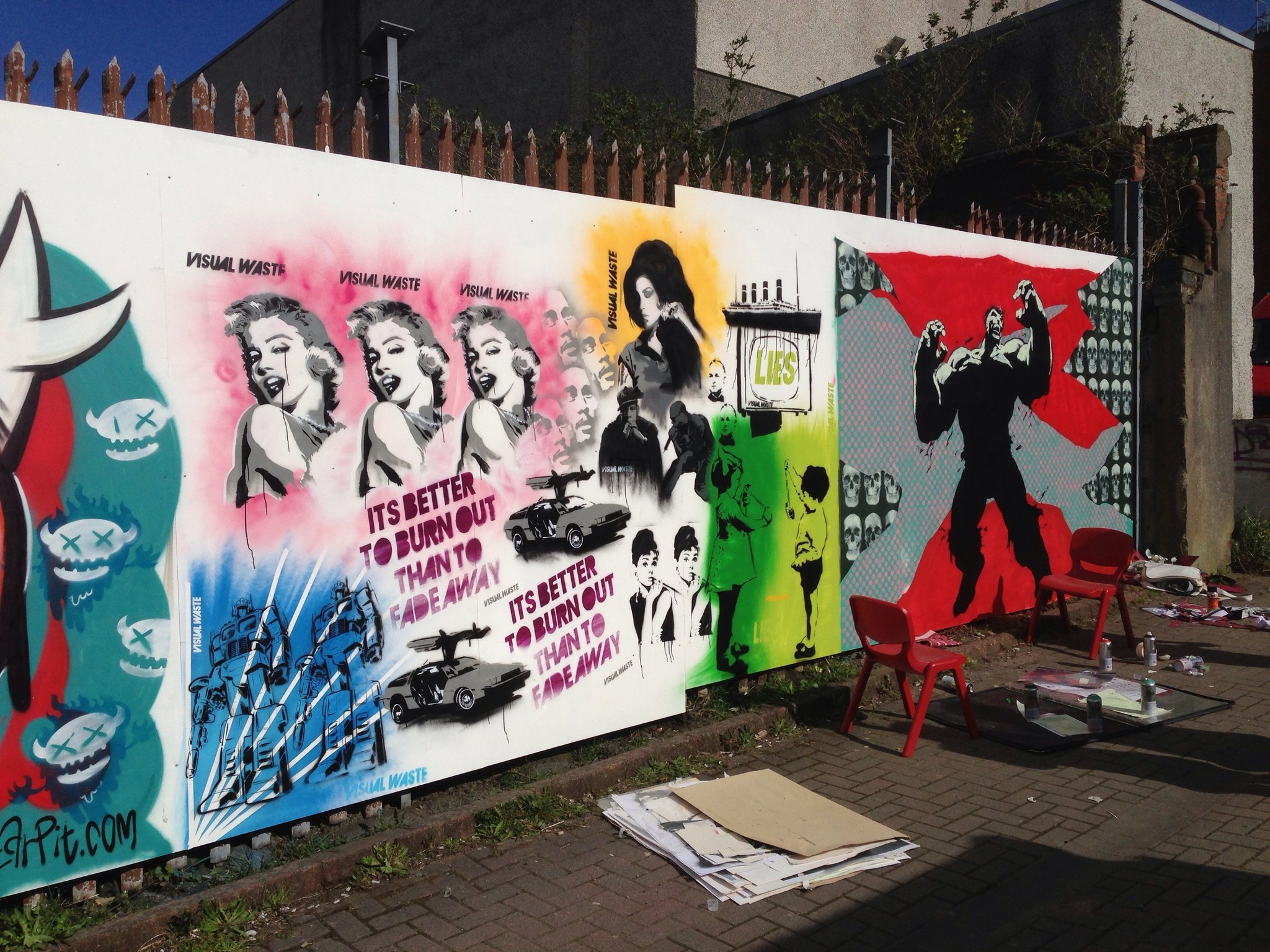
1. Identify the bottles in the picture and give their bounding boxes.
[1140,678,1156,716]
[1024,683,1039,721]
[941,675,974,695]
[1086,693,1104,733]
[1099,638,1112,671]
[1207,585,1218,612]
[1143,631,1157,666]
[1173,655,1205,672]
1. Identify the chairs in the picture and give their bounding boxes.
[1027,528,1136,660]
[839,595,980,757]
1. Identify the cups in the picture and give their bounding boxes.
[707,899,718,911]
